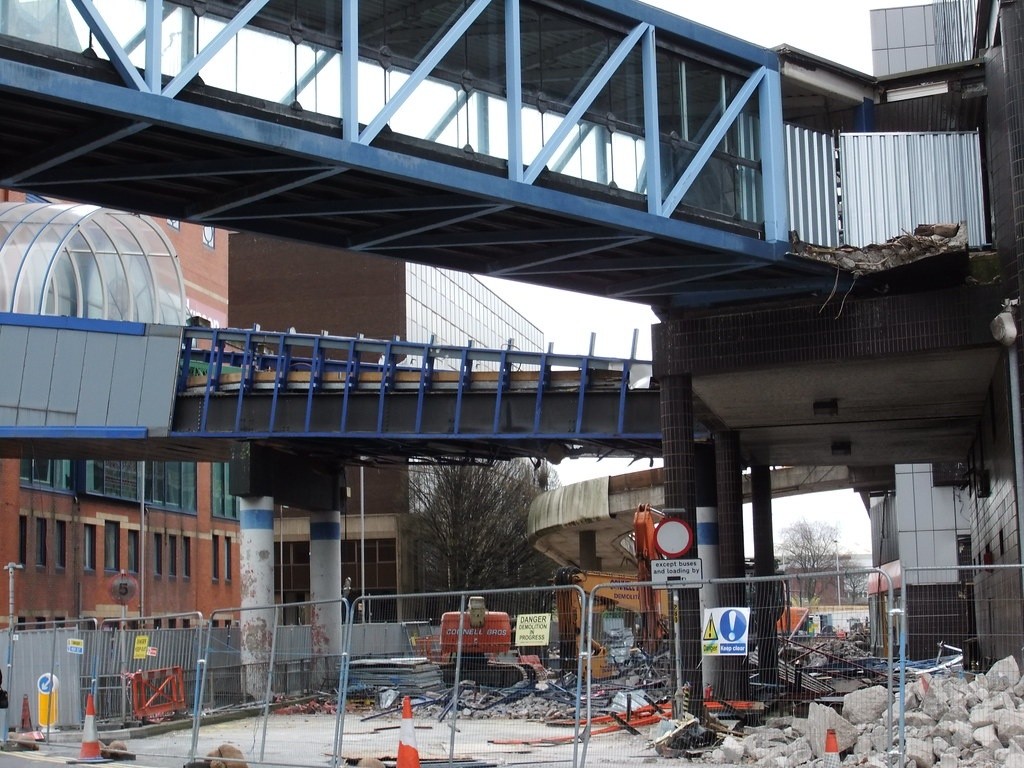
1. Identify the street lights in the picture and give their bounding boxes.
[833,539,841,604]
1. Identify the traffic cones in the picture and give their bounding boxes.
[9,694,43,741]
[66,692,113,764]
[821,726,841,768]
[394,695,421,768]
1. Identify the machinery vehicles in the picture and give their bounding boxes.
[554,565,670,681]
[432,596,546,686]
[633,502,810,654]
